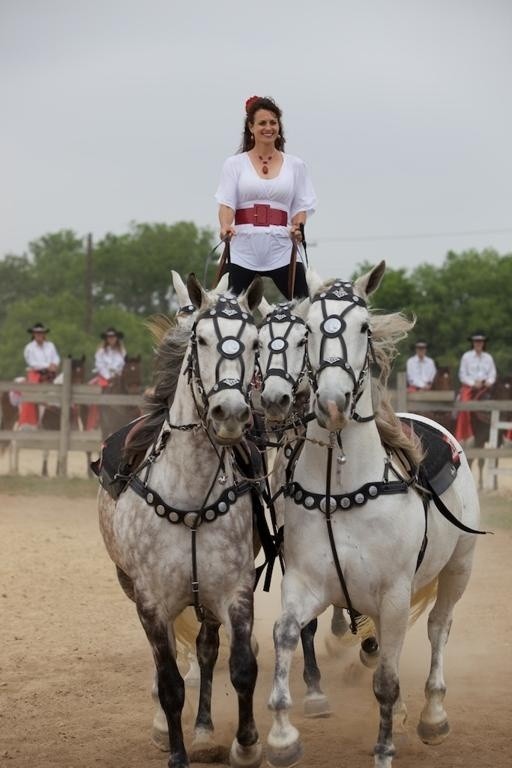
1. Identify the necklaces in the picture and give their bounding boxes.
[253,148,276,175]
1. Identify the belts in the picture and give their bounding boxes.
[236,204,286,228]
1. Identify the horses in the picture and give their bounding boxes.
[0,352,86,476]
[96,258,482,768]
[80,352,148,479]
[454,368,511,491]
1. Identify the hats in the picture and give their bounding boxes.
[410,340,430,351]
[27,323,49,333]
[468,330,488,342]
[101,328,123,340]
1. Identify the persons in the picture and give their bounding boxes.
[212,94,320,304]
[85,326,127,431]
[405,337,437,393]
[450,329,498,422]
[15,320,60,433]
